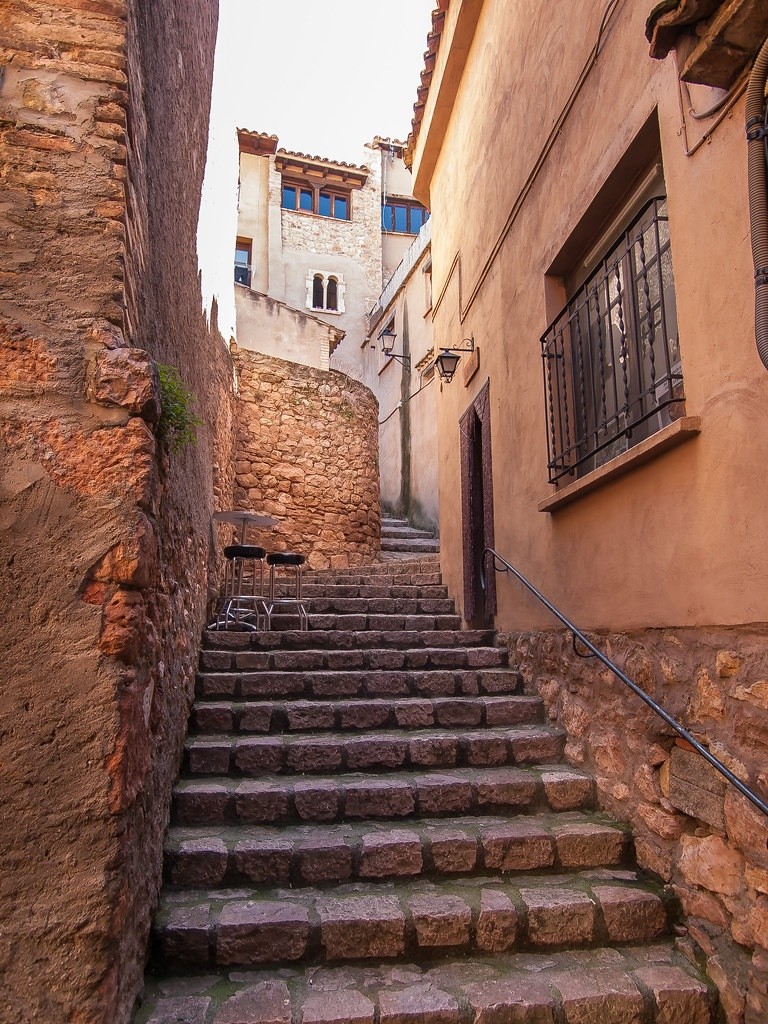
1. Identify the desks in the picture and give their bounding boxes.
[208,511,279,632]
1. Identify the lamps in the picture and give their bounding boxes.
[377,328,411,377]
[434,336,474,383]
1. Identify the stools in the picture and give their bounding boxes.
[263,551,309,631]
[216,543,271,631]
